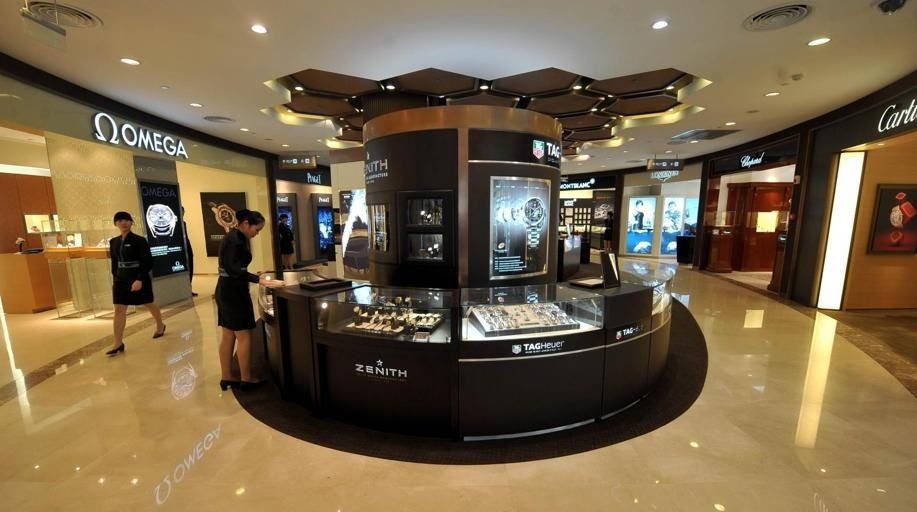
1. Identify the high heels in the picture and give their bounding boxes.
[106,344,124,354]
[152,324,166,338]
[219,378,262,390]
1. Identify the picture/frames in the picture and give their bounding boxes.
[865,183,916,255]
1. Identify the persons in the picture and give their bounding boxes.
[214,209,281,392]
[664,201,680,230]
[604,211,614,254]
[182,206,199,296]
[106,211,166,355]
[279,214,296,270]
[632,200,644,230]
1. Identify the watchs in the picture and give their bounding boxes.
[479,303,573,330]
[521,197,547,251]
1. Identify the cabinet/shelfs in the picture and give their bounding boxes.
[704,225,734,273]
